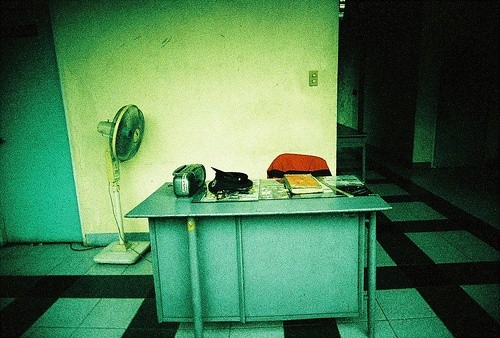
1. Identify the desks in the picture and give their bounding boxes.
[124,175,392,338]
[337,122,366,185]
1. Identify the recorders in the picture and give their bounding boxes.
[172,163,205,197]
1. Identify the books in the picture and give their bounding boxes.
[283,174,324,194]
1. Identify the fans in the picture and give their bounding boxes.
[93,104,151,264]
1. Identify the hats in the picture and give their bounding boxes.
[208,166,253,193]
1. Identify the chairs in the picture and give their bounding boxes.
[267,153,332,178]
